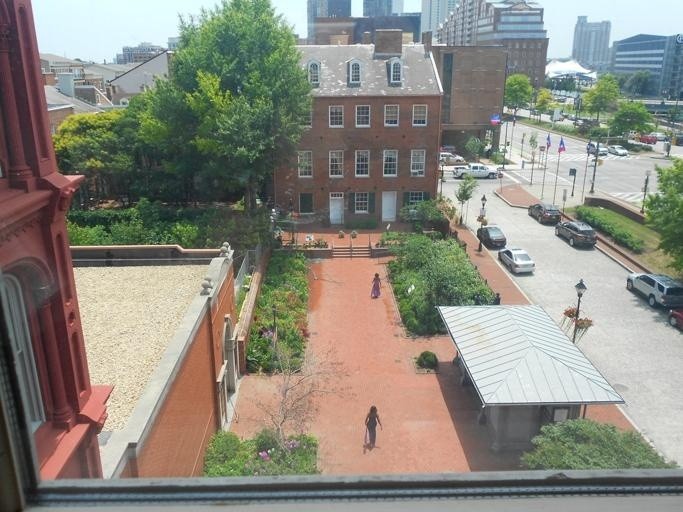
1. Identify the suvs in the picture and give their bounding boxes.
[626,272,683,311]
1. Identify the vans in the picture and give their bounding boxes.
[586,141,609,155]
[439,152,466,166]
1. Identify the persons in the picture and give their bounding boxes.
[474,293,481,305]
[494,293,500,304]
[364,405,383,452]
[370,272,381,298]
[629,130,641,142]
[664,131,678,145]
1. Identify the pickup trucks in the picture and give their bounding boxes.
[453,162,498,180]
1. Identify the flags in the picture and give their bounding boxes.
[557,136,567,154]
[545,131,552,152]
[489,113,500,126]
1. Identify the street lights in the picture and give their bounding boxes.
[572,278,587,343]
[478,194,487,252]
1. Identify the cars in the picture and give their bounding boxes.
[498,247,536,275]
[628,130,683,146]
[668,307,683,333]
[528,203,562,224]
[555,220,598,249]
[608,144,629,156]
[508,102,600,126]
[477,226,506,249]
